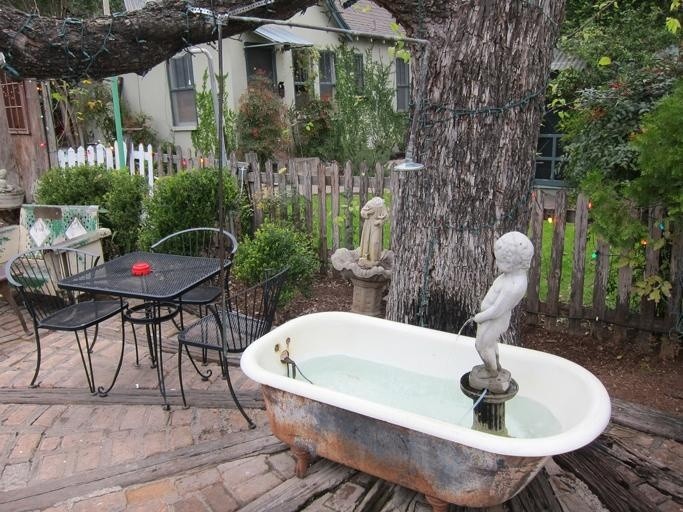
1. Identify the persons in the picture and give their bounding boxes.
[247,63,273,86]
[359,197,389,260]
[474,232,532,378]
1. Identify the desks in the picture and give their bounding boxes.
[56,249,231,411]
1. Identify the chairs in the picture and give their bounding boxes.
[4,246,141,396]
[175,263,294,429]
[148,225,240,368]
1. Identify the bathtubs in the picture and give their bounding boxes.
[240,310,611,512]
